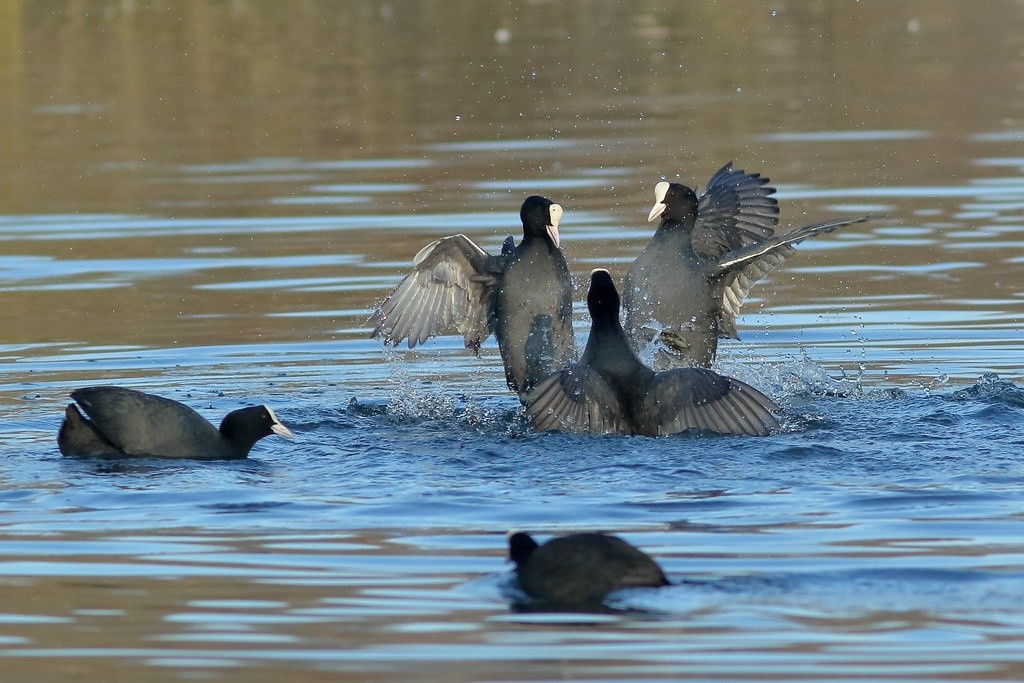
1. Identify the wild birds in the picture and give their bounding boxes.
[504,531,678,603]
[369,160,870,437]
[58,385,294,461]
